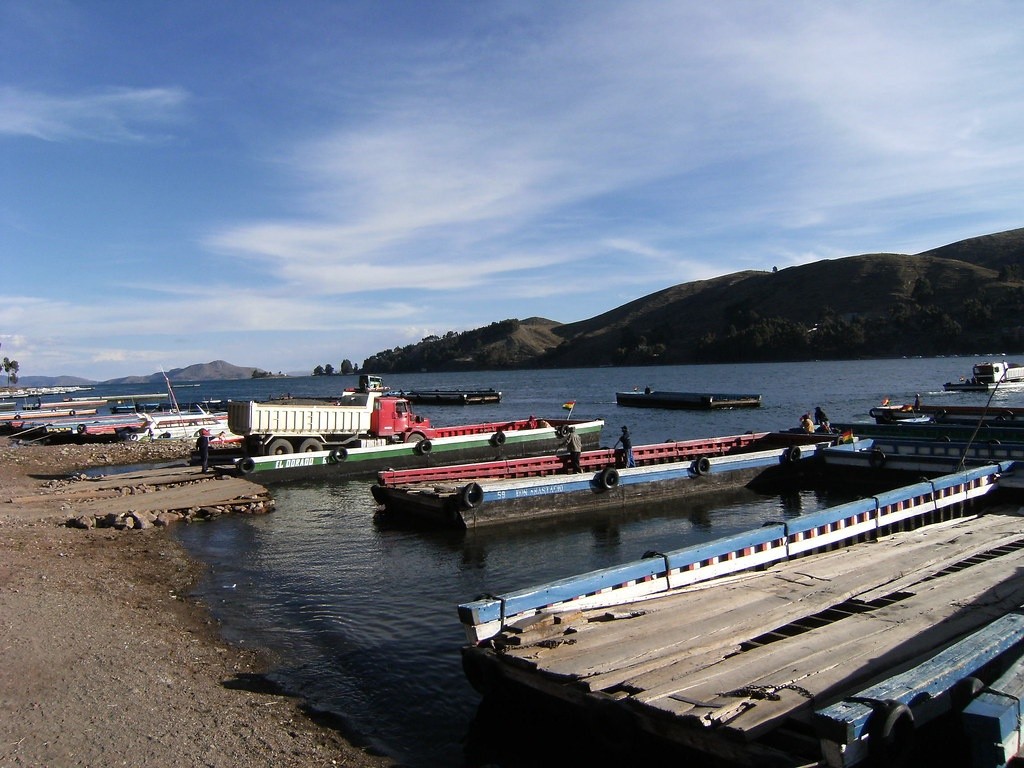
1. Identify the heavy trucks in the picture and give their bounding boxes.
[227,388,433,457]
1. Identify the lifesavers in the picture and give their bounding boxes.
[333,446,348,464]
[493,433,507,447]
[419,439,432,455]
[602,468,620,491]
[77,423,88,434]
[462,483,485,507]
[873,703,920,766]
[70,410,76,416]
[787,445,801,464]
[239,458,255,475]
[869,451,887,469]
[697,455,711,477]
[15,414,21,420]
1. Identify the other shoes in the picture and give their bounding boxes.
[202,470,208,473]
[572,472,578,474]
[581,469,583,474]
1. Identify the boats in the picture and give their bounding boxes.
[615,385,764,413]
[389,385,504,406]
[367,431,841,525]
[0,393,228,441]
[194,415,607,483]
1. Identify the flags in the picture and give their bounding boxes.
[842,429,854,444]
[881,395,890,406]
[562,401,576,411]
[902,404,912,411]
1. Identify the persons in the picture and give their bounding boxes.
[219,431,226,444]
[196,430,210,473]
[915,393,921,410]
[559,427,583,474]
[644,385,650,395]
[799,407,830,433]
[619,426,636,468]
[148,425,154,443]
[525,415,537,429]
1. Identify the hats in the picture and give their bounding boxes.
[814,407,821,411]
[621,425,627,430]
[802,414,811,419]
[917,394,920,396]
[201,429,209,435]
